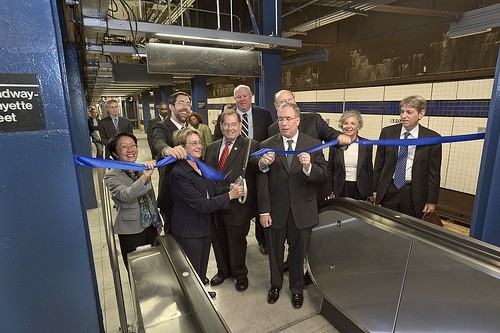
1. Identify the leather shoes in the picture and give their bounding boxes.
[267,287,280,304]
[292,293,304,308]
[234,278,249,292]
[210,273,233,286]
[283,261,289,273]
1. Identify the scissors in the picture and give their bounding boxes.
[233,137,252,204]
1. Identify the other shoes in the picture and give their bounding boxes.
[208,291,216,298]
[257,242,267,255]
[303,274,313,285]
[204,277,209,285]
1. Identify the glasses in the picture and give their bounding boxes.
[185,142,201,146]
[173,102,190,106]
[116,144,137,151]
[277,117,297,124]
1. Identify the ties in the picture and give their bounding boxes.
[241,114,248,139]
[114,119,119,130]
[393,132,411,190]
[286,140,294,169]
[219,141,233,176]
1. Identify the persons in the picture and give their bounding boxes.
[188,112,213,160]
[328,109,374,205]
[148,92,192,237]
[88,109,103,159]
[169,127,245,297]
[148,101,170,159]
[258,102,330,309]
[204,110,259,291]
[213,85,272,256]
[268,89,352,286]
[104,132,163,273]
[373,95,442,220]
[99,100,133,208]
